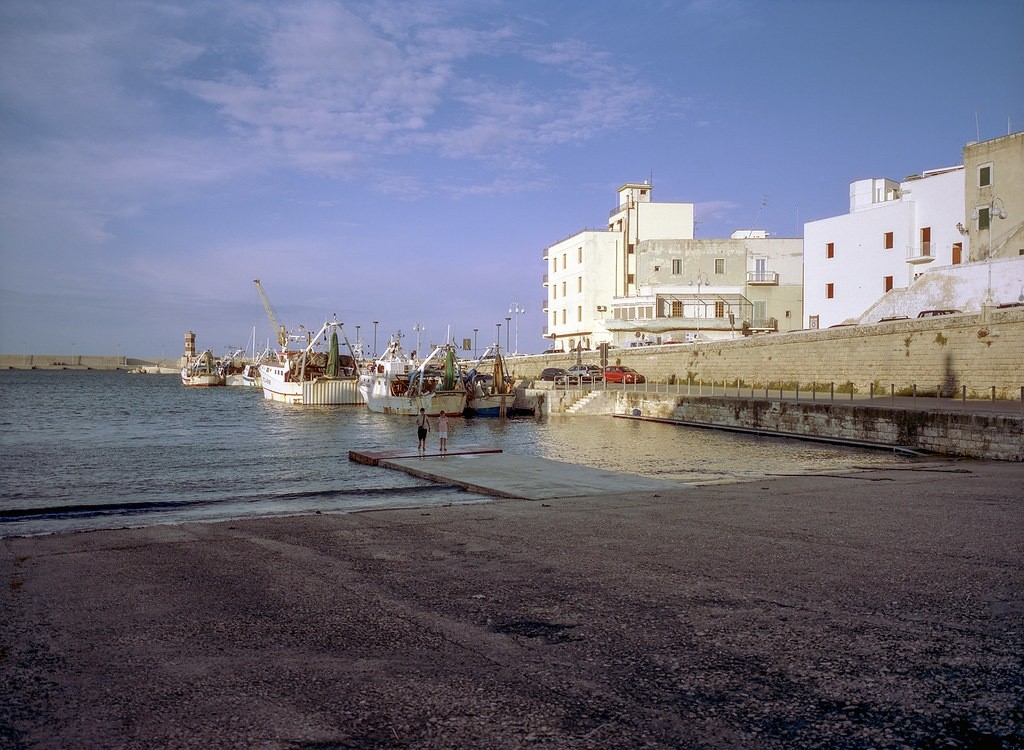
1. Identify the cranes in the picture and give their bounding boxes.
[253,279,288,347]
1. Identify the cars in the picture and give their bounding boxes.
[878,309,963,323]
[569,363,602,380]
[597,340,683,350]
[602,366,645,383]
[569,348,592,352]
[541,367,579,385]
[542,349,565,354]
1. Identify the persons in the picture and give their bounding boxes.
[463,403,471,423]
[437,410,448,451]
[416,408,431,449]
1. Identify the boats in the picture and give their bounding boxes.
[180,326,270,387]
[257,312,371,404]
[356,330,516,416]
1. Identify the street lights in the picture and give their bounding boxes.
[509,301,525,356]
[970,194,1008,301]
[373,321,379,358]
[505,317,511,352]
[355,325,360,344]
[688,265,710,339]
[472,328,478,360]
[495,323,501,346]
[413,320,426,358]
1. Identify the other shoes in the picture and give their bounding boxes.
[439,448,442,451]
[418,445,421,449]
[444,448,448,452]
[423,447,426,450]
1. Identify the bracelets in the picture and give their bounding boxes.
[429,428,431,429]
